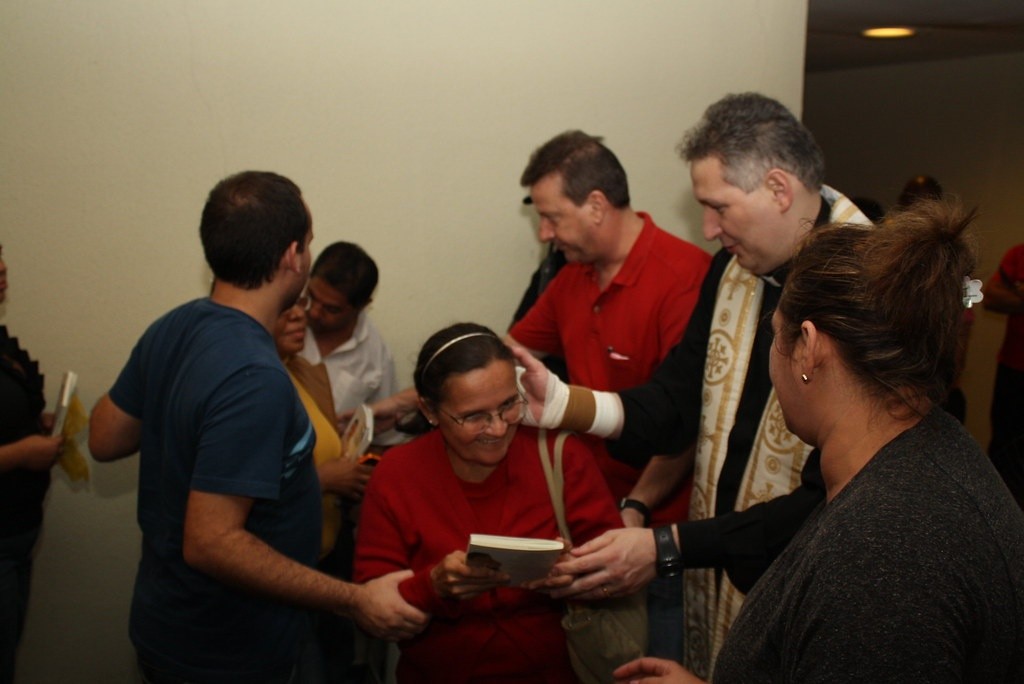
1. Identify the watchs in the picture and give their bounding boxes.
[651,526,682,577]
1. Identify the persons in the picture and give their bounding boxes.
[607,197,1024,684]
[502,130,711,663]
[275,240,408,684]
[0,240,70,684]
[894,171,976,428]
[355,321,625,684]
[554,91,878,684]
[966,239,1024,510]
[87,170,427,684]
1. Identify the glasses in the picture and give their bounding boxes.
[429,388,529,435]
[296,296,312,310]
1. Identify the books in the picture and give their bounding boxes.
[339,405,373,463]
[50,368,79,437]
[464,531,564,587]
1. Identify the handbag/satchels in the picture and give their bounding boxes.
[537,427,649,684]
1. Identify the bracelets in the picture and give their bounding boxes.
[616,495,652,523]
